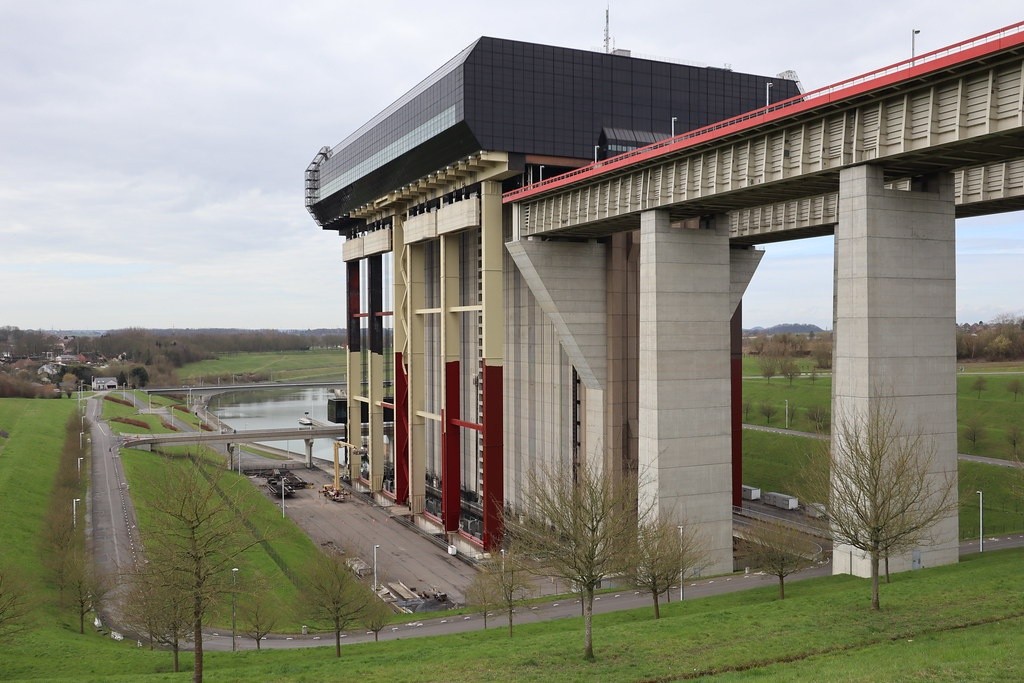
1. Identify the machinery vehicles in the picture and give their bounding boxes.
[319,441,358,502]
